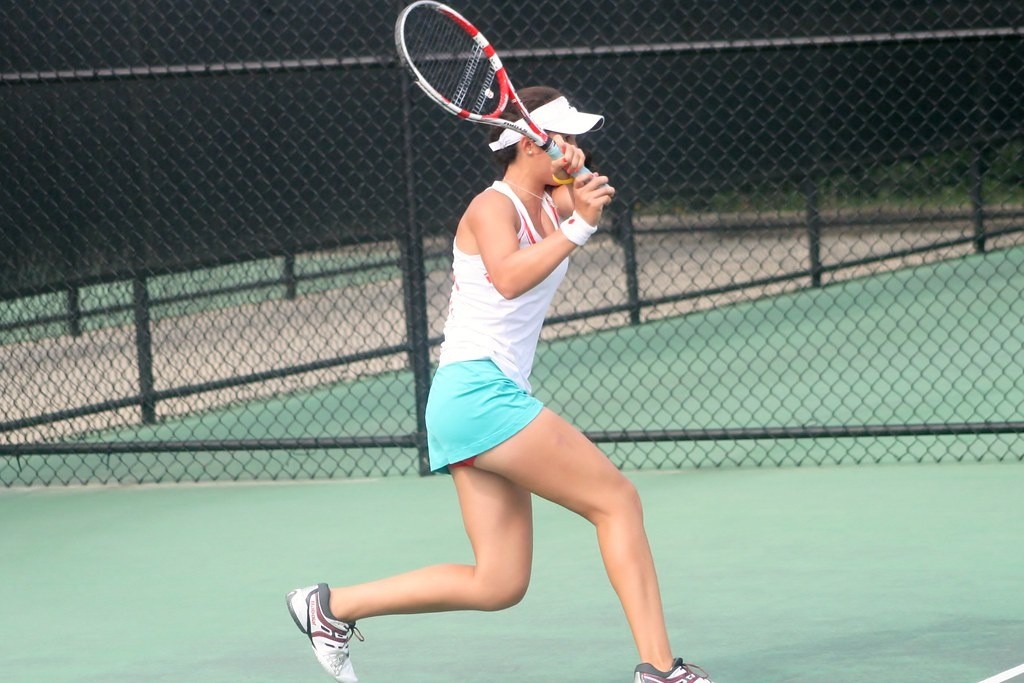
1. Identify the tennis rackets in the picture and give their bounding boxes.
[394,0,611,191]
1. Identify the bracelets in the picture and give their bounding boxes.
[553,171,574,184]
[561,210,597,245]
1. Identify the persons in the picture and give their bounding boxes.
[287,87,710,683]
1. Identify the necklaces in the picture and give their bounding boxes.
[502,177,550,200]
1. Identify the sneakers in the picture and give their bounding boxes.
[286,582,364,683]
[634,658,714,683]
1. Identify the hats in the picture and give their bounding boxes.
[488,96,605,152]
